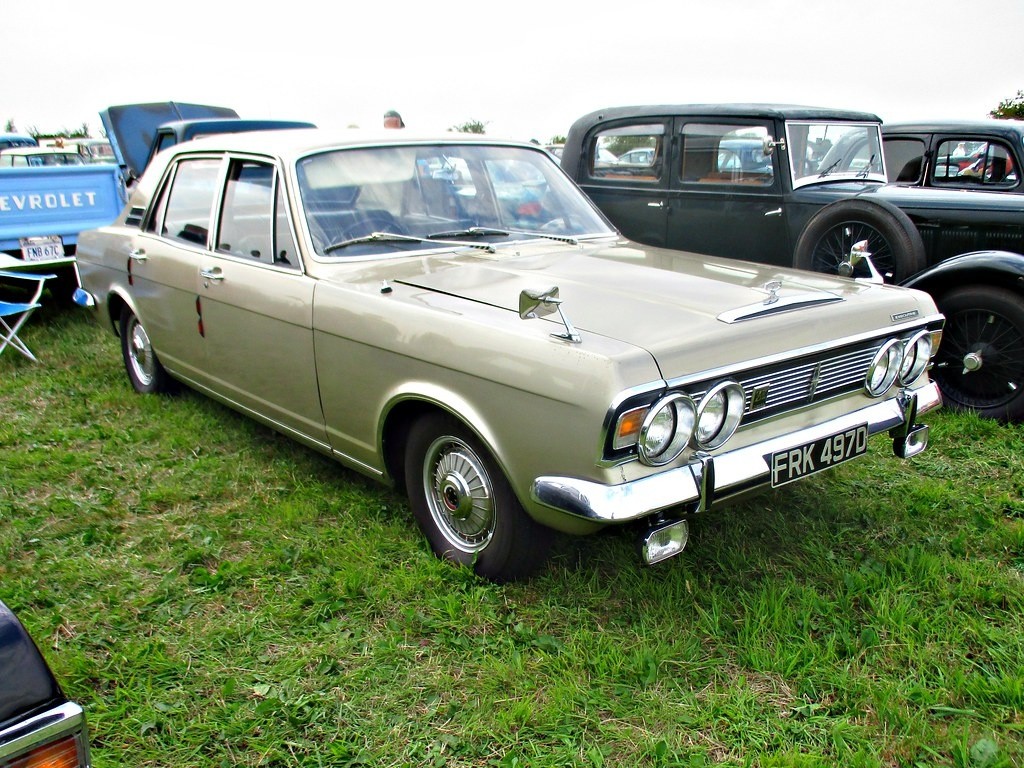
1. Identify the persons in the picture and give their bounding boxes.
[384,110,405,128]
[953,142,965,156]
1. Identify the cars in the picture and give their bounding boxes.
[932,136,1022,187]
[808,121,1024,201]
[705,136,793,181]
[0,597,93,767]
[95,95,370,242]
[553,100,1023,436]
[72,126,949,577]
[0,143,87,174]
[613,147,660,170]
[539,141,618,172]
[422,141,563,235]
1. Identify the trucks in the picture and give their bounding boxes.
[0,158,130,321]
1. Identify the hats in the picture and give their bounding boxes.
[384,110,406,127]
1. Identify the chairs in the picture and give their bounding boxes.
[1,265,56,367]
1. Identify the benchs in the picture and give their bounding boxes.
[168,208,403,268]
